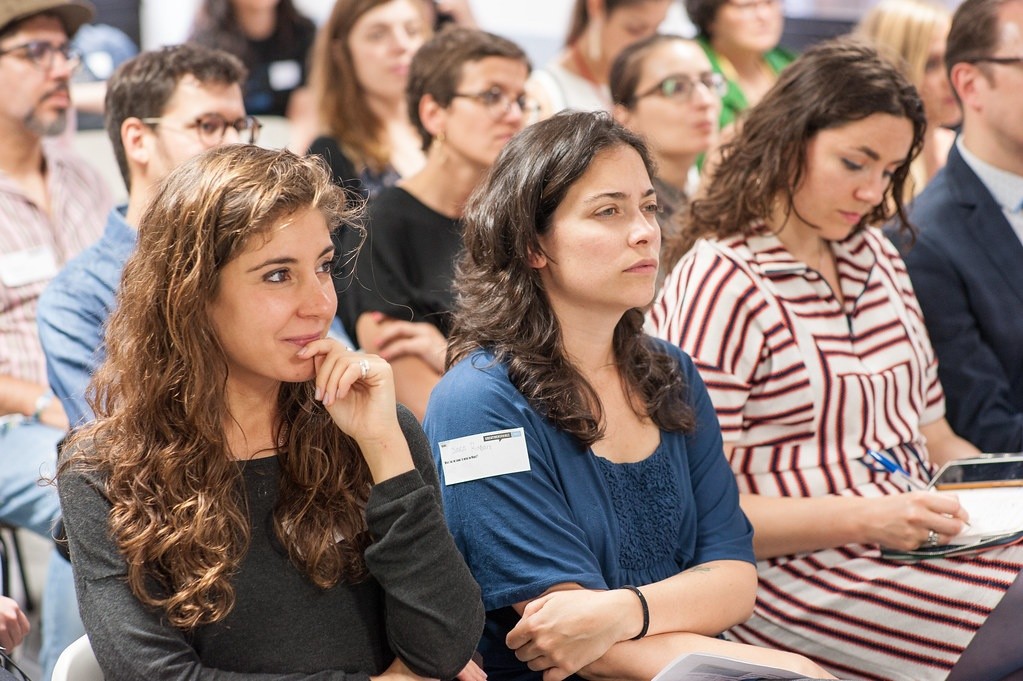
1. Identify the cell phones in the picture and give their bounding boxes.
[934,459,1023,490]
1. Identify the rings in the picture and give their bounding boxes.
[926,533,937,547]
[358,357,369,381]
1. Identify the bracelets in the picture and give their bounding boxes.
[29,389,56,424]
[621,584,649,642]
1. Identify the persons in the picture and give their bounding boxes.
[0,0,1023,681]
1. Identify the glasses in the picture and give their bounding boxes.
[142,112,262,149]
[453,86,530,114]
[1,42,69,66]
[632,74,726,103]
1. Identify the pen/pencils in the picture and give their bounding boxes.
[866,449,972,528]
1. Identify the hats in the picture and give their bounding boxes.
[0,0,94,39]
